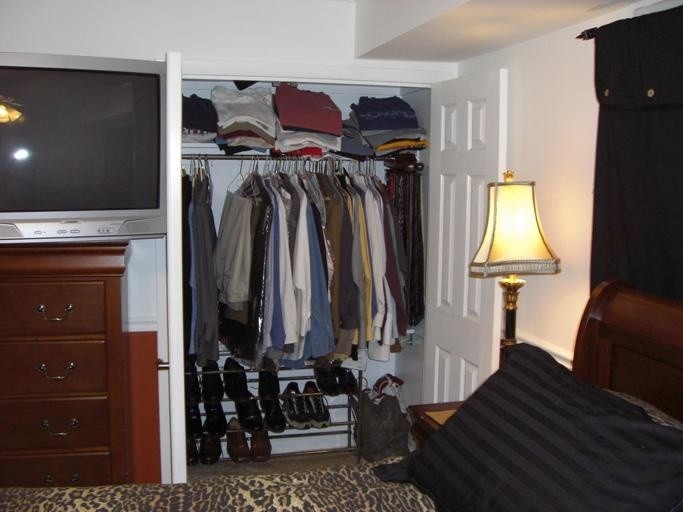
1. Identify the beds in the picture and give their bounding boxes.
[1,277,681,512]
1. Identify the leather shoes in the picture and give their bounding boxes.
[185,357,356,463]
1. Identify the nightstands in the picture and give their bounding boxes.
[407,401,463,453]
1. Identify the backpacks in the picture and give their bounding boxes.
[355,389,409,460]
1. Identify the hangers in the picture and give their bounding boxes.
[181,154,385,204]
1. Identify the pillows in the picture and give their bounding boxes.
[371,343,682,511]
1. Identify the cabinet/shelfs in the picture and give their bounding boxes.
[1,241,133,488]
[184,352,362,466]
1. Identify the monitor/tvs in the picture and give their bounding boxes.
[0,53,166,243]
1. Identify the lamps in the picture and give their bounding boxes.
[468,170,559,368]
